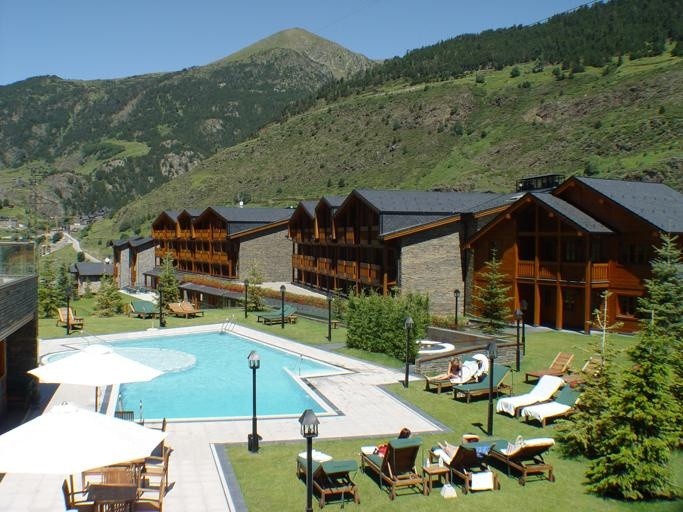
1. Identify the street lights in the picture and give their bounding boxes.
[158,283,165,326]
[280,285,286,329]
[244,280,249,319]
[403,316,414,388]
[299,410,320,512]
[454,289,461,324]
[486,299,529,435]
[66,288,72,334]
[248,351,261,451]
[326,293,333,342]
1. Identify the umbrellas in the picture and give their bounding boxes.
[0,344,165,505]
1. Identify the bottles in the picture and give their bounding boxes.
[439,456,444,467]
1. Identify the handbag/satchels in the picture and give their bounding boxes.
[506,435,526,455]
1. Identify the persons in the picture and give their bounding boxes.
[424,353,490,387]
[376,427,412,459]
[437,433,479,459]
[521,297,528,323]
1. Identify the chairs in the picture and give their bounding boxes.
[424,350,605,429]
[127,300,204,321]
[295,437,556,509]
[255,304,297,325]
[62,410,171,512]
[54,306,83,331]
